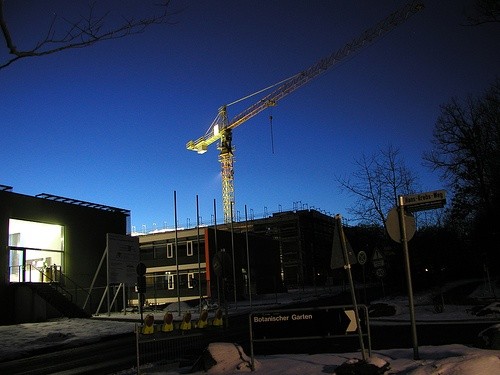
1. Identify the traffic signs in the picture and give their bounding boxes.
[250,306,368,341]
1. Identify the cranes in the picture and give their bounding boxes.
[183,0,428,224]
